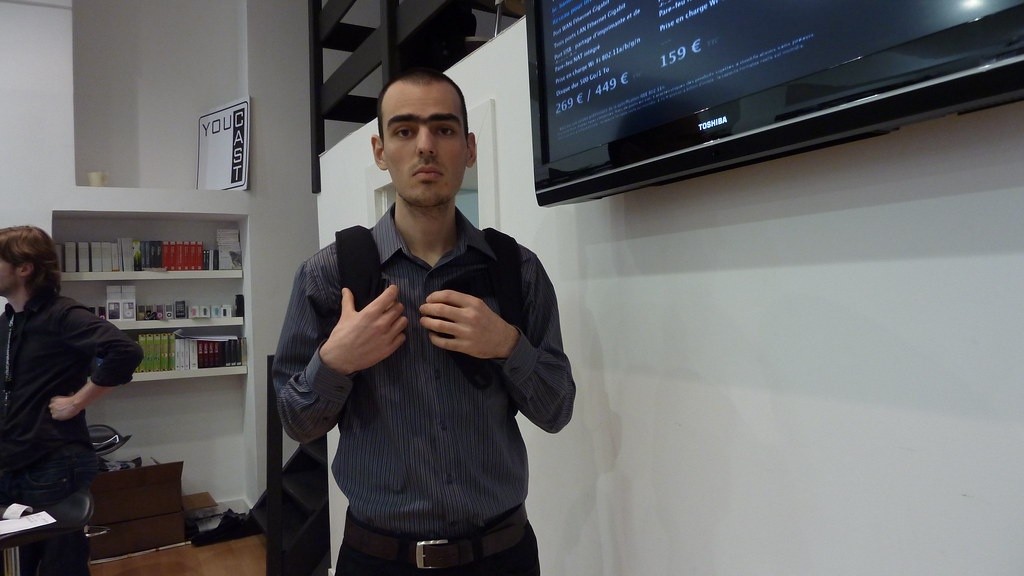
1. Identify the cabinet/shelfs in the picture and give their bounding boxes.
[52,210,248,383]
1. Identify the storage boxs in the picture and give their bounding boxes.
[182,491,217,519]
[87,460,219,561]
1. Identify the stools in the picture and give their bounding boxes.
[0,508,82,576]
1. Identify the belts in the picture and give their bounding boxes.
[344,503,525,570]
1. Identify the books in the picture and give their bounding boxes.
[135,329,247,373]
[53,229,242,273]
[185,491,223,519]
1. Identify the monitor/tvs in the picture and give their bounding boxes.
[526,0,1024,207]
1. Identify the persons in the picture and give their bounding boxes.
[269,73,575,576]
[0,225,144,576]
